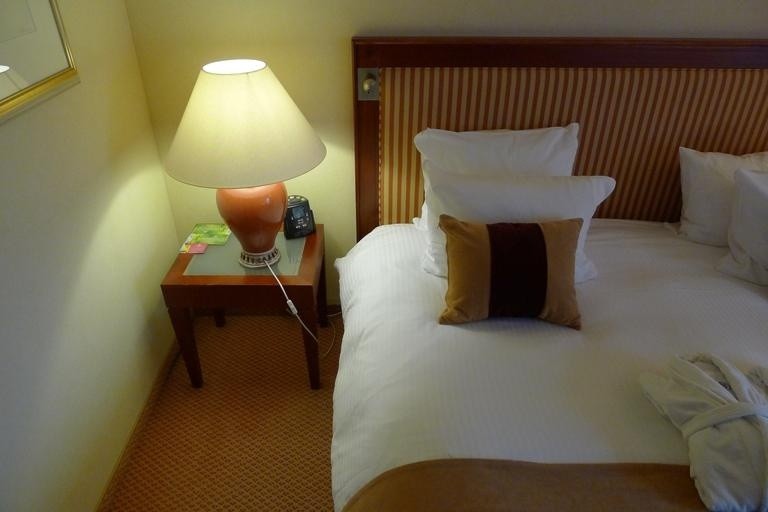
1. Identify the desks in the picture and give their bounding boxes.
[160,222,330,390]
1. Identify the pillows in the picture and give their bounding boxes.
[435,212,585,332]
[676,145,768,245]
[712,166,768,290]
[421,160,617,285]
[411,121,580,237]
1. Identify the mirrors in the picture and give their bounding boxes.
[1,0,82,129]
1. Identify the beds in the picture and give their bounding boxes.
[329,35,766,511]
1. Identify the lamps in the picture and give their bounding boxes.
[163,58,327,271]
